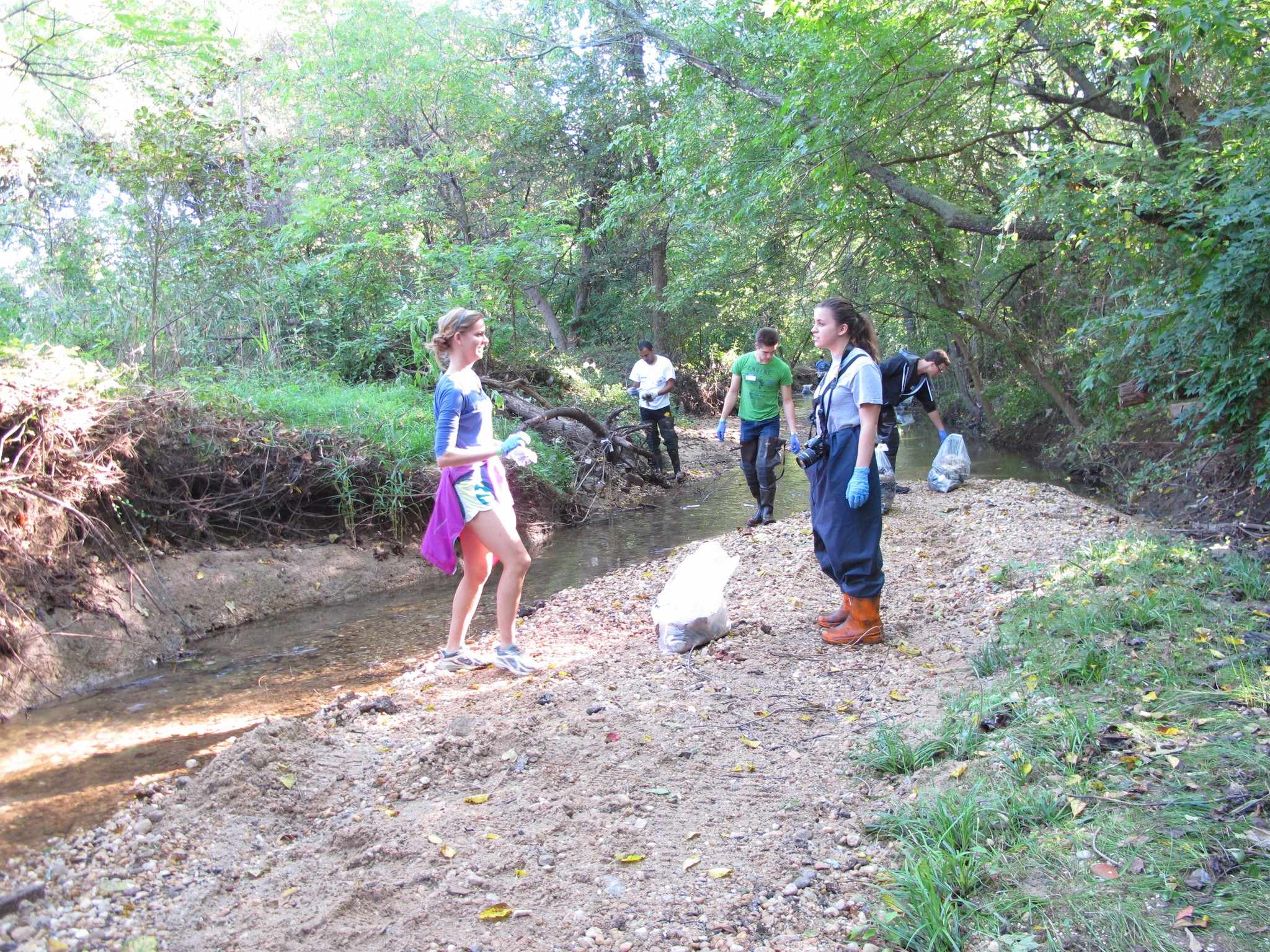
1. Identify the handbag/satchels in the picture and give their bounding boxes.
[802,384,813,394]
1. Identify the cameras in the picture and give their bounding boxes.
[796,438,831,470]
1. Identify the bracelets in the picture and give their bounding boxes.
[720,418,726,422]
[790,433,798,436]
[495,447,502,455]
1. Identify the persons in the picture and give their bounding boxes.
[717,327,800,527]
[423,307,542,677]
[815,356,830,385]
[627,341,685,484]
[804,295,885,644]
[877,348,952,494]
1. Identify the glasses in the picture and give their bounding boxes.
[932,360,944,374]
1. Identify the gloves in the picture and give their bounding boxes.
[501,431,531,457]
[789,434,800,455]
[846,467,870,509]
[717,421,725,442]
[509,448,537,466]
[642,391,658,403]
[627,387,637,397]
[939,430,948,444]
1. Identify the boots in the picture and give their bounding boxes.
[651,449,667,482]
[667,449,687,483]
[745,486,762,526]
[820,595,885,645]
[818,593,851,628]
[759,488,776,525]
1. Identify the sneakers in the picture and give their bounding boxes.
[497,644,539,676]
[437,644,492,671]
[896,484,910,494]
